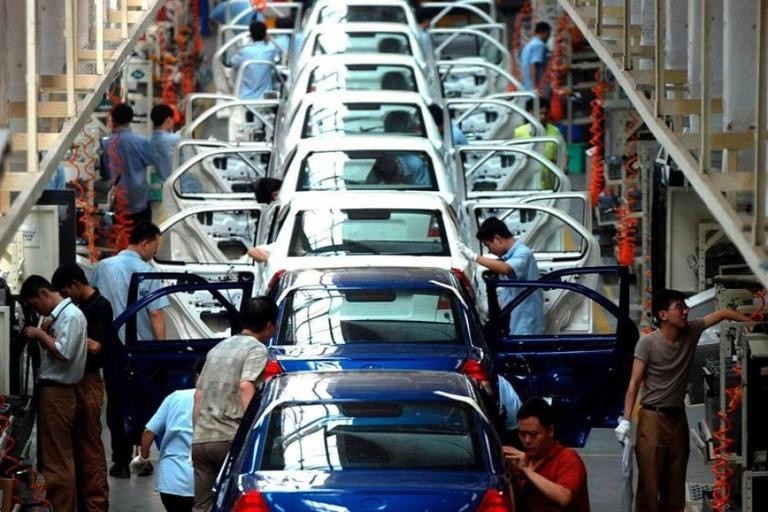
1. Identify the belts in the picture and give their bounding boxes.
[642,404,665,412]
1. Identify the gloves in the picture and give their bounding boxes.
[128,455,149,474]
[457,241,477,262]
[614,420,630,442]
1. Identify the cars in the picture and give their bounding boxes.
[211,370,520,511]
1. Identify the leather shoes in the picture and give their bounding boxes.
[109,465,130,478]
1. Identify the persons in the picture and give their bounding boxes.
[40,264,113,512]
[514,97,568,190]
[498,375,526,452]
[519,22,553,99]
[191,295,280,512]
[373,153,432,186]
[500,396,590,512]
[87,222,170,479]
[456,217,546,336]
[416,104,469,162]
[128,355,205,512]
[415,10,434,57]
[20,275,89,512]
[614,290,752,512]
